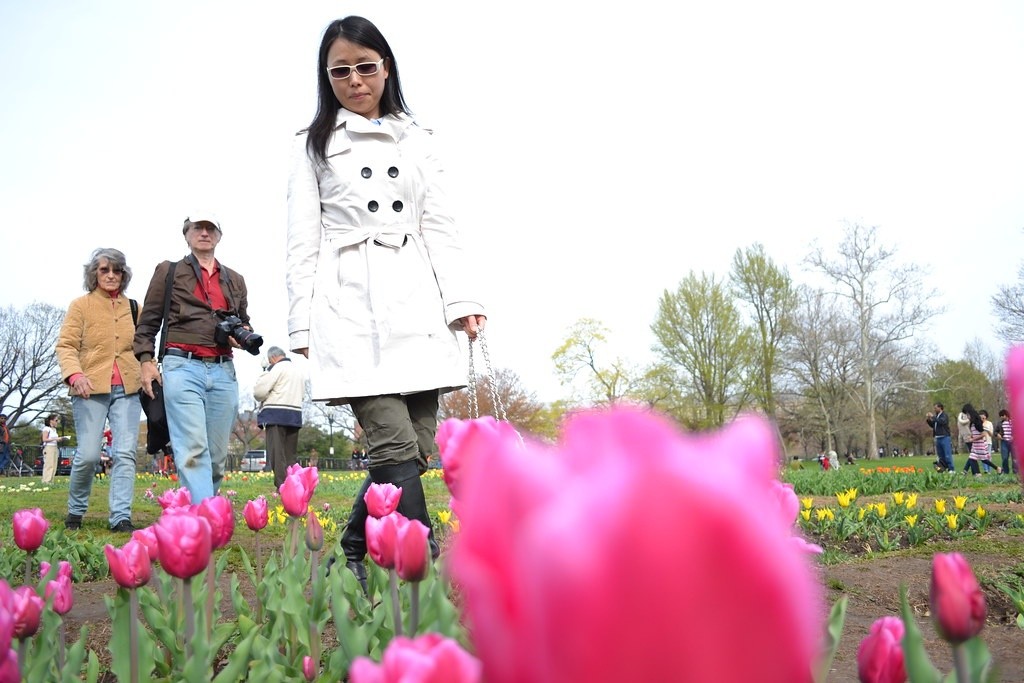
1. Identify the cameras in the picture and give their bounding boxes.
[214,315,264,355]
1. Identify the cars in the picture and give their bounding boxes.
[240,450,267,473]
[33,446,104,476]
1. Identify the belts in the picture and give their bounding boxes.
[164,348,232,363]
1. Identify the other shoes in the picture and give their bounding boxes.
[111,520,136,534]
[975,473,982,477]
[996,467,1004,476]
[64,513,82,530]
[948,470,955,474]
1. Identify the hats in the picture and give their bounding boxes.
[184,209,221,230]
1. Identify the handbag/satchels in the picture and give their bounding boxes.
[138,373,171,455]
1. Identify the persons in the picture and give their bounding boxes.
[994,410,1018,474]
[957,404,1004,475]
[56,249,157,531]
[979,409,993,473]
[818,448,830,470]
[310,448,318,467]
[893,448,909,457]
[879,445,886,457]
[42,415,62,484]
[792,456,804,470]
[254,346,302,491]
[0,414,11,473]
[927,451,931,455]
[845,452,854,464]
[287,16,489,592]
[133,210,254,506]
[103,429,112,457]
[926,402,954,473]
[351,448,368,470]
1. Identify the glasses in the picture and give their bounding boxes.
[326,57,383,80]
[189,223,217,234]
[96,267,125,275]
[1,417,7,420]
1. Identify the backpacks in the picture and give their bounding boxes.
[39,427,50,450]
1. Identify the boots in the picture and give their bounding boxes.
[367,461,440,559]
[326,474,379,595]
